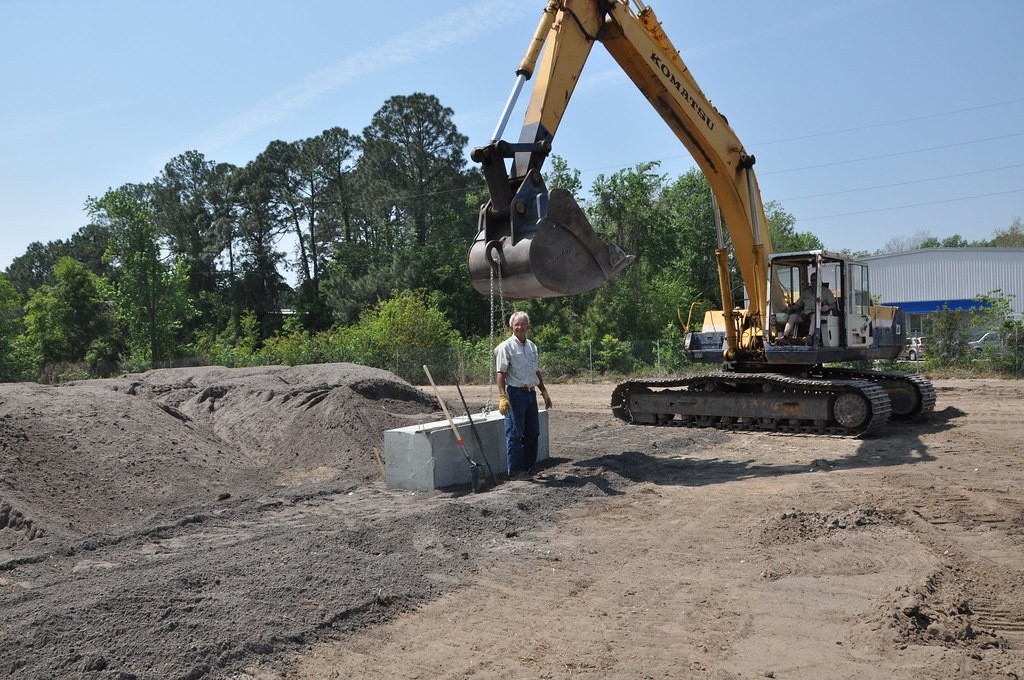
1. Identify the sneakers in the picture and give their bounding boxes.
[802,336,813,346]
[775,337,788,345]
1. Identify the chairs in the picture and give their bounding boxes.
[804,282,832,321]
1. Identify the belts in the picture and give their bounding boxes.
[508,385,536,393]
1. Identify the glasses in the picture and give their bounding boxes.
[811,278,817,282]
[512,324,529,329]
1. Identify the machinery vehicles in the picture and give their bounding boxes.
[468,0,938,439]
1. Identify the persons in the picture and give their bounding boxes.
[493,312,552,480]
[774,273,837,345]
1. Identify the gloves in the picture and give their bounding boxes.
[541,390,553,410]
[498,395,511,416]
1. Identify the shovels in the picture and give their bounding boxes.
[423,365,490,494]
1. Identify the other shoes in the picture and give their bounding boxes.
[509,471,533,480]
[527,468,538,476]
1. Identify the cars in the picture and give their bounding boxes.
[901,335,928,360]
[968,332,1001,358]
